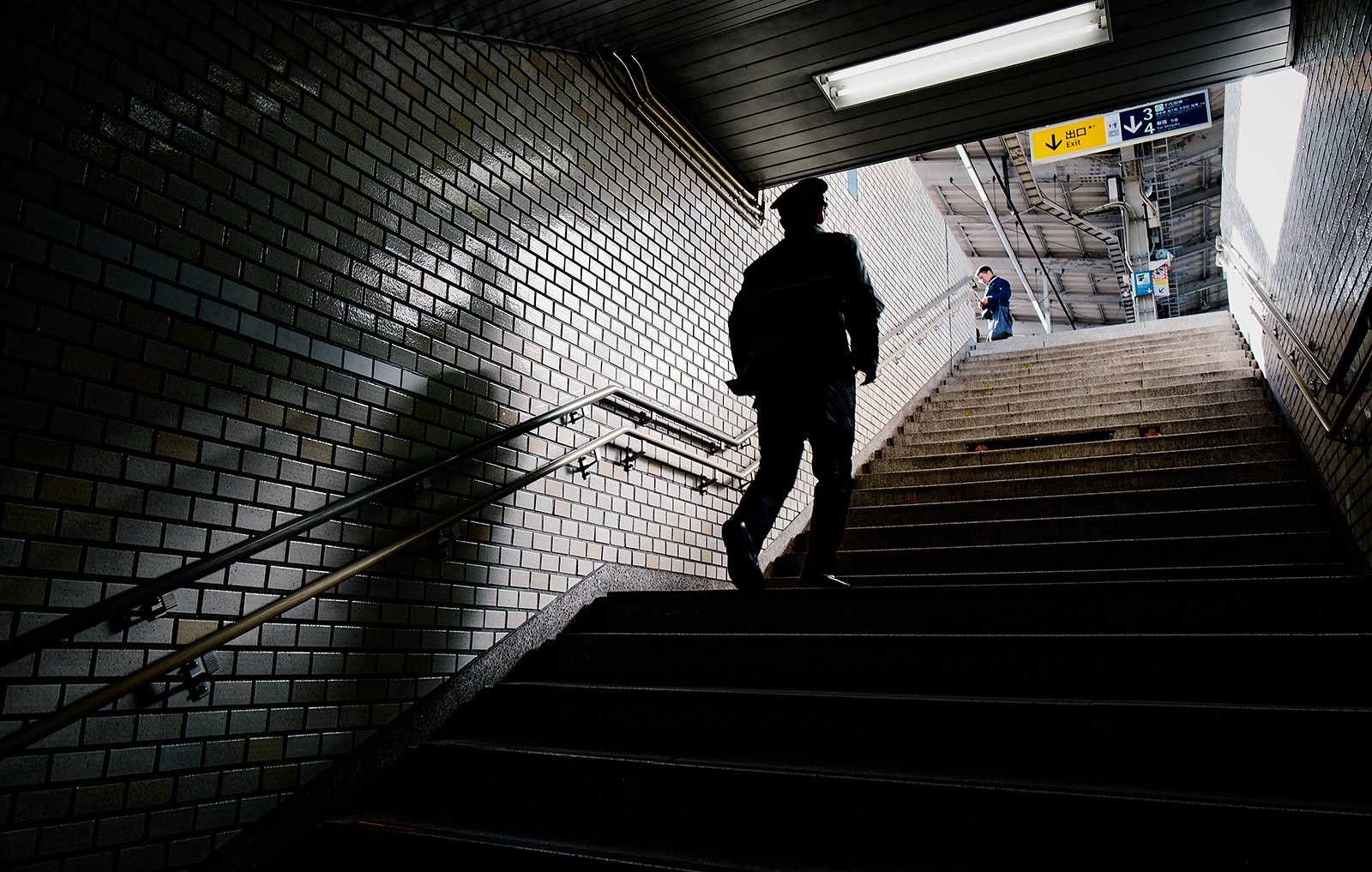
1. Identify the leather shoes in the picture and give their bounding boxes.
[723,518,765,590]
[800,567,853,587]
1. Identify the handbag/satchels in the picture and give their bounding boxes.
[991,294,1012,340]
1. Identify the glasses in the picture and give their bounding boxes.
[821,206,830,216]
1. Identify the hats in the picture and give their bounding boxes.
[771,176,829,211]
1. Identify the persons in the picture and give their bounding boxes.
[718,175,888,590]
[974,264,1014,342]
